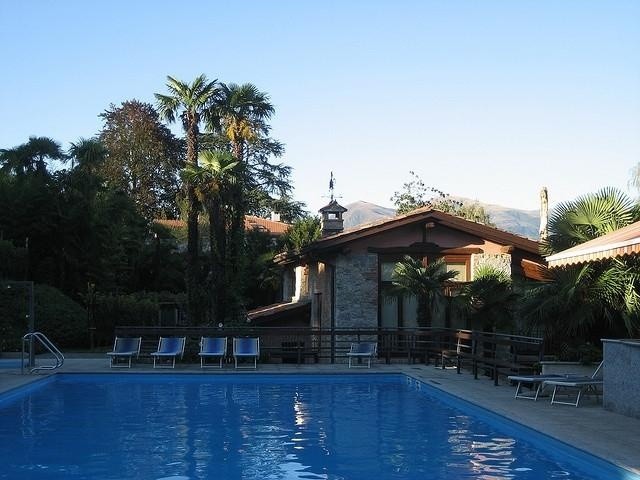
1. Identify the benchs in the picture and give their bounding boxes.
[425,330,544,386]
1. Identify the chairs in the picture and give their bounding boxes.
[150,337,186,369]
[107,337,142,369]
[507,359,605,407]
[233,338,261,370]
[197,338,227,369]
[347,344,379,370]
